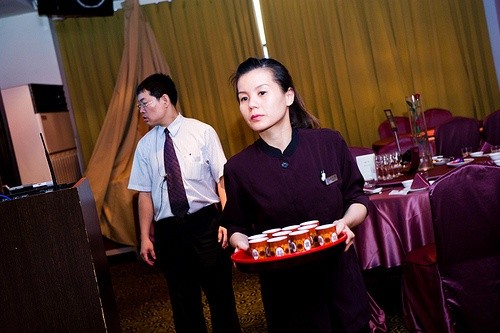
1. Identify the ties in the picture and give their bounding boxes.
[163,128,190,219]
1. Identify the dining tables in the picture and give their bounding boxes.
[350,157,500,273]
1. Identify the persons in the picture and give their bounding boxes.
[223,57,372,333]
[127,72,242,333]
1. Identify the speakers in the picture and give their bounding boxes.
[37,0,114,18]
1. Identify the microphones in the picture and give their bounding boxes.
[40,133,60,190]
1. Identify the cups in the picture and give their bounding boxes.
[375,151,404,181]
[262,228,281,239]
[248,233,268,241]
[282,224,300,231]
[298,224,318,247]
[272,231,292,253]
[248,237,269,258]
[300,220,320,226]
[461,147,472,158]
[289,229,312,253]
[314,224,339,245]
[266,236,291,257]
[491,146,500,153]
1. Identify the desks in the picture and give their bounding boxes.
[0,177,121,333]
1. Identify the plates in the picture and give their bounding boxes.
[363,186,382,194]
[404,181,434,192]
[470,152,500,166]
[432,158,450,165]
[447,158,475,166]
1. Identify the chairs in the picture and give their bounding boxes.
[480,108,500,152]
[348,145,377,159]
[418,106,454,131]
[378,135,419,156]
[377,114,410,140]
[434,115,483,155]
[402,162,500,333]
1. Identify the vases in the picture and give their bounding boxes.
[408,106,433,162]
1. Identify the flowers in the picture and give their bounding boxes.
[405,92,425,142]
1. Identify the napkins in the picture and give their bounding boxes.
[411,169,430,189]
[477,139,495,153]
[363,179,376,190]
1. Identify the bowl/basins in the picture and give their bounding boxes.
[402,179,413,188]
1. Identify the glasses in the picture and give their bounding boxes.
[135,98,157,110]
